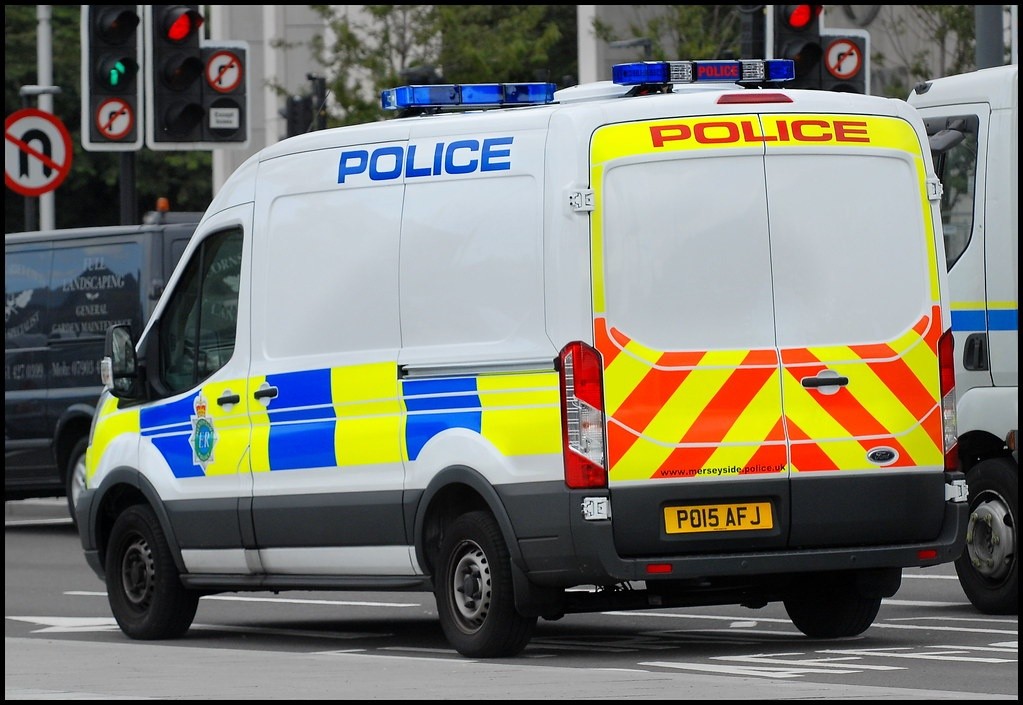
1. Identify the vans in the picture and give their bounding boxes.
[897,64,1023,618]
[5,195,235,508]
[75,59,975,660]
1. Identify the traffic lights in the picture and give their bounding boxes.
[765,3,825,91]
[821,27,871,99]
[80,4,147,152]
[201,40,247,144]
[144,5,210,150]
